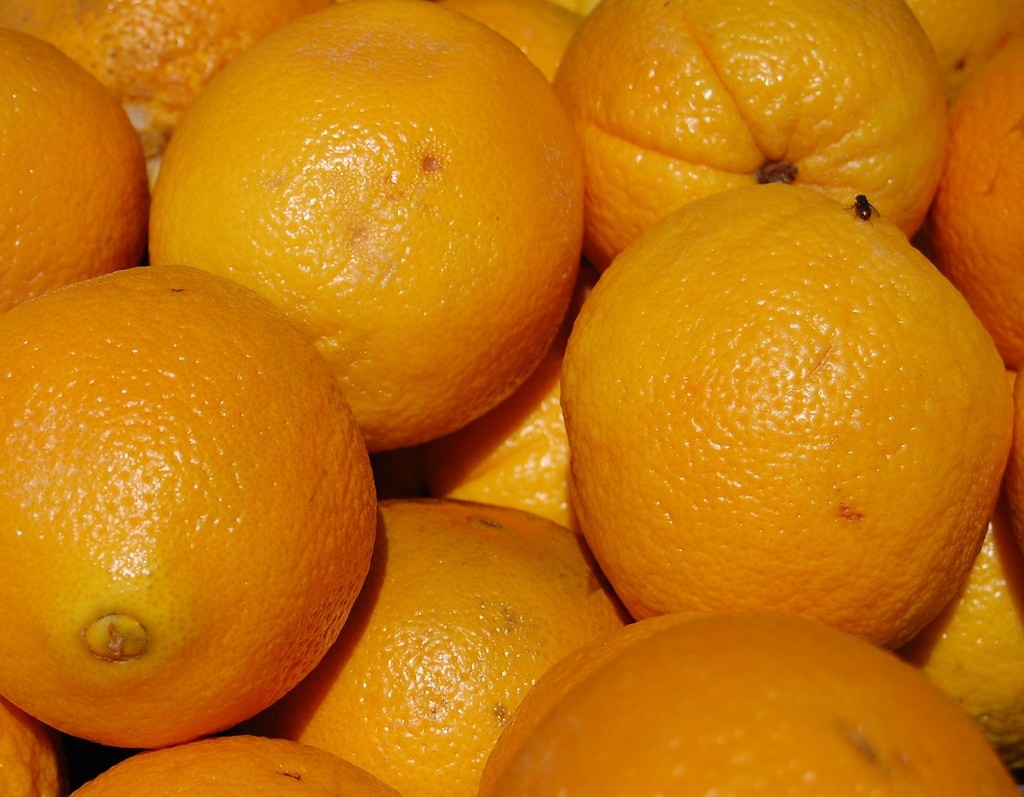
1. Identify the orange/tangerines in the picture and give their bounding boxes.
[1,2,1024,797]
